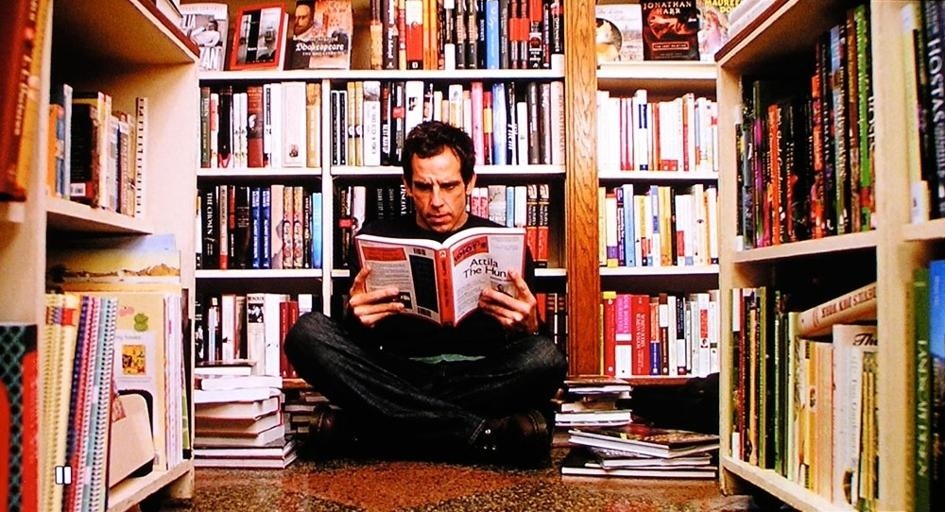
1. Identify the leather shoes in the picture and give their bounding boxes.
[309,403,354,445]
[486,409,547,452]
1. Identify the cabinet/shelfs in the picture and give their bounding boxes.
[198,66,568,390]
[0,0,201,512]
[596,64,721,387]
[715,0,944,512]
